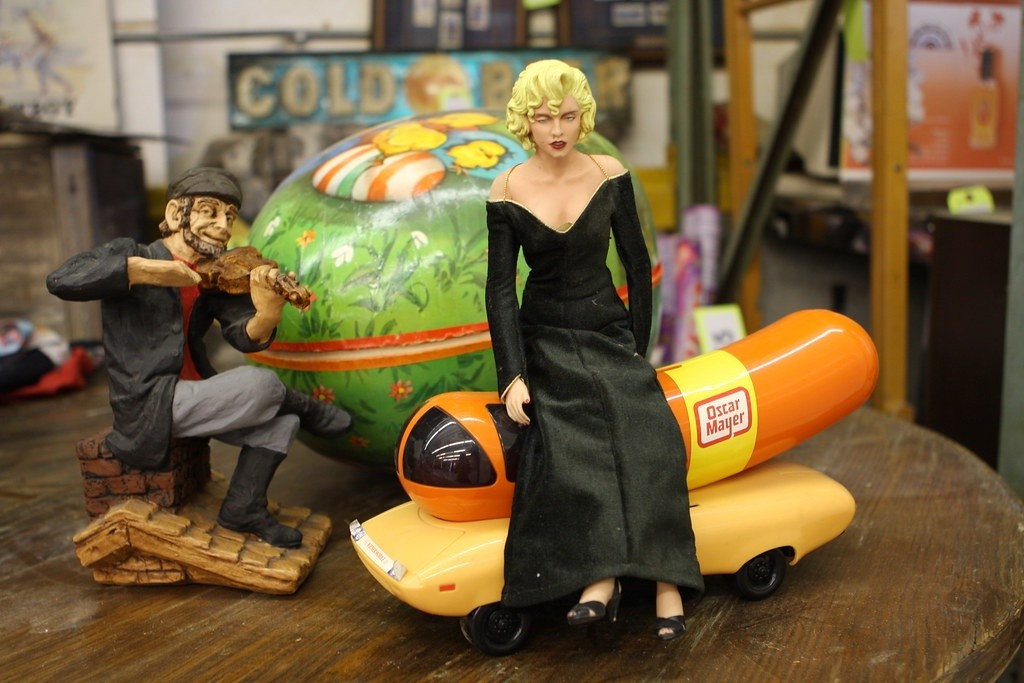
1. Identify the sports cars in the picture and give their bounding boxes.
[346,463,856,658]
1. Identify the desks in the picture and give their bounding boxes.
[0,342,1023,681]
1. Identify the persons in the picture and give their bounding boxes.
[45,166,354,549]
[485,58,706,645]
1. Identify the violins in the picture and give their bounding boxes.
[194,246,311,309]
